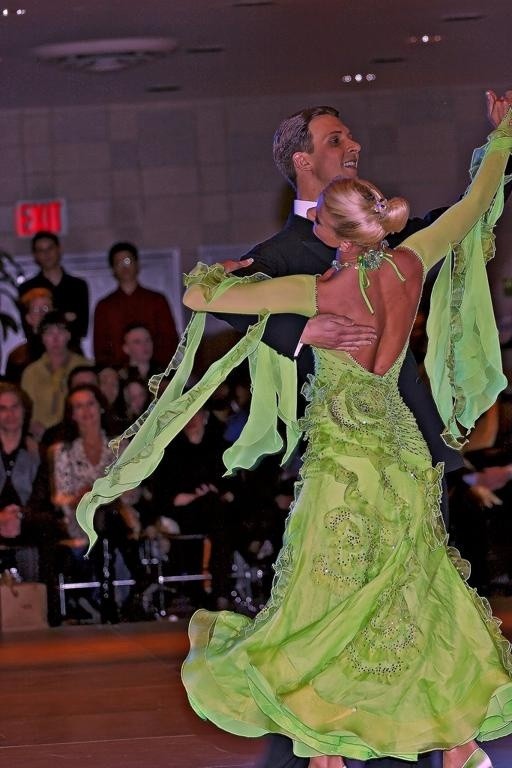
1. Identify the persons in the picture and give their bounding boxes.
[411,288,510,601]
[20,231,90,351]
[92,241,180,370]
[0,288,313,627]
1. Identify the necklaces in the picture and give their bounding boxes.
[330,240,388,273]
